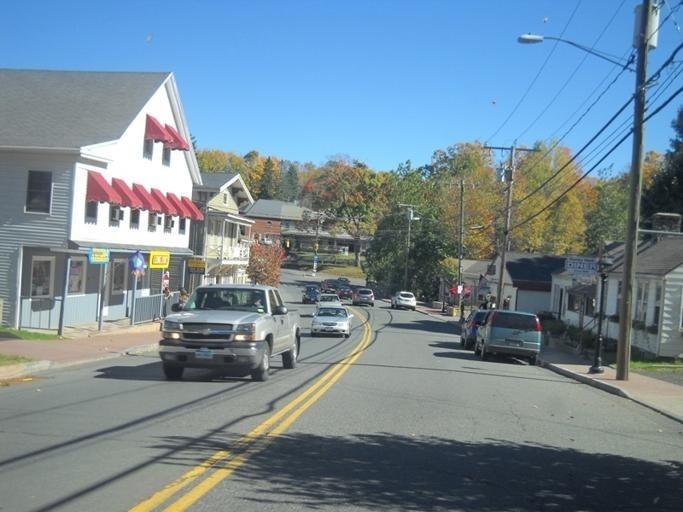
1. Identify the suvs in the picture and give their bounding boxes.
[474,309,541,366]
[458,310,486,350]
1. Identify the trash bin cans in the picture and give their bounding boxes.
[448,307,456,316]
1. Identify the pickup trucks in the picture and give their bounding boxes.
[155,283,302,383]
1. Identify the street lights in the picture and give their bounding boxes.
[516,28,641,385]
[587,249,614,375]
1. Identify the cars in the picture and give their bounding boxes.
[389,291,417,312]
[309,306,354,338]
[301,276,376,311]
